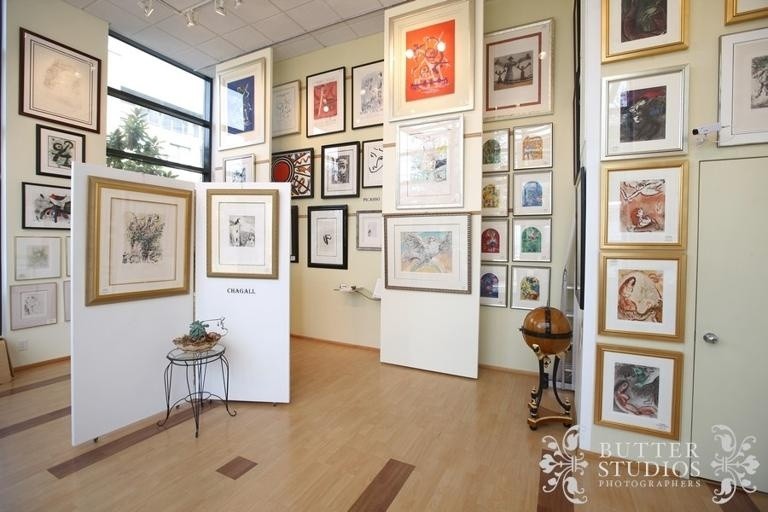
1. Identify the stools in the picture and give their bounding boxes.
[157,346,238,440]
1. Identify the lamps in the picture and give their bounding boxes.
[136,0,241,27]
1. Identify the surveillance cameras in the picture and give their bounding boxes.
[691,122,722,136]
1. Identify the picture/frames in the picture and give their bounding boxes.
[597,0,692,67]
[596,154,693,250]
[593,341,687,440]
[597,62,692,162]
[10,23,197,334]
[724,0,767,23]
[593,247,689,344]
[712,26,767,149]
[204,0,559,315]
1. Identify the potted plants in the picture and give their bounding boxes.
[174,320,221,352]
